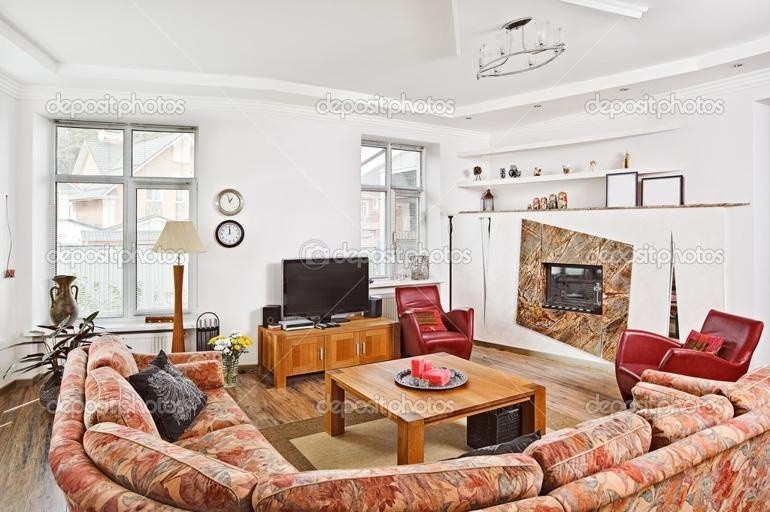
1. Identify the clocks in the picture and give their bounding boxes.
[216,219,244,250]
[214,188,245,217]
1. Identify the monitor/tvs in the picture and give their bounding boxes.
[283,257,369,322]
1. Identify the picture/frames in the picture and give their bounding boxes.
[640,174,684,207]
[604,171,640,208]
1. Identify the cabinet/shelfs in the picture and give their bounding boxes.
[456,125,687,189]
[258,324,324,387]
[325,316,399,370]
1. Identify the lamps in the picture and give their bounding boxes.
[435,201,460,312]
[149,216,208,353]
[475,14,569,81]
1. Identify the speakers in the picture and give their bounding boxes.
[364,297,382,318]
[263,305,281,328]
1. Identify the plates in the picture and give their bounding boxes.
[395,367,466,390]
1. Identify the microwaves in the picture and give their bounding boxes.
[545,264,603,313]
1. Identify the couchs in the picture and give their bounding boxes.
[394,280,475,361]
[49,332,768,510]
[614,309,764,410]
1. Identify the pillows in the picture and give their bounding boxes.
[436,428,543,463]
[681,328,725,358]
[122,348,209,441]
[411,307,449,335]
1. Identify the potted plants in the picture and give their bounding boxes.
[2,306,108,416]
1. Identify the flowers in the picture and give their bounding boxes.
[206,329,252,357]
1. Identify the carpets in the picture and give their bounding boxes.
[259,403,583,472]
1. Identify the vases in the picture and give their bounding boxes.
[48,274,81,330]
[220,356,239,387]
